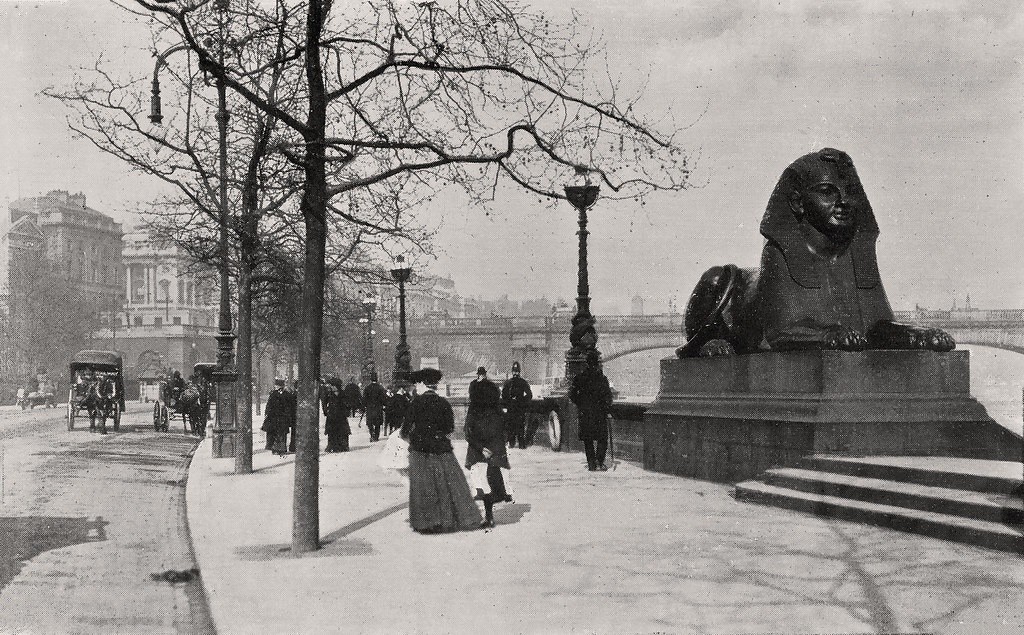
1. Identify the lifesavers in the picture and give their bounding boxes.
[547,410,561,452]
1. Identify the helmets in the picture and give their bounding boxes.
[512,362,520,371]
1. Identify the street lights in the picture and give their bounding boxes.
[547,163,621,398]
[144,45,235,459]
[387,253,417,390]
[360,292,378,385]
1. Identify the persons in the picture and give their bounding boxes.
[568,352,614,473]
[29,361,536,455]
[399,367,484,531]
[464,388,516,527]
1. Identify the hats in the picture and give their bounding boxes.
[476,367,487,374]
[274,379,285,386]
[421,368,443,385]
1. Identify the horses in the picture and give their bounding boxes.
[85,372,121,436]
[180,382,210,437]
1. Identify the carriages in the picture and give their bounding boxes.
[190,362,219,402]
[152,379,211,438]
[68,349,126,434]
